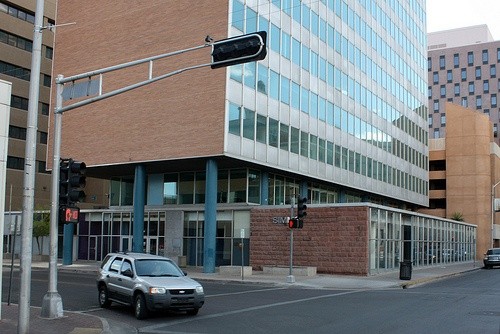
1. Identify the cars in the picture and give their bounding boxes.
[482,248,500,269]
[95,250,204,320]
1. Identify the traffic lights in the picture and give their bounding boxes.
[297,194,308,220]
[59,157,71,207]
[59,206,81,223]
[66,159,88,207]
[288,218,297,228]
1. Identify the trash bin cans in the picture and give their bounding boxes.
[400,262,412,280]
